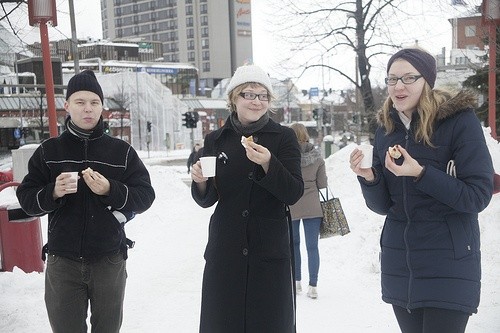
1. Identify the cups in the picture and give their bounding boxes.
[61,172,78,193]
[199,157,217,177]
[356,145,373,169]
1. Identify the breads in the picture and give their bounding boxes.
[388,145,401,159]
[244,135,253,148]
[82,167,93,177]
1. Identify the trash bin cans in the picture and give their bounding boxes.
[0,181,44,273]
[11,143,41,190]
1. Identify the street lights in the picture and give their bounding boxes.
[27,1,58,138]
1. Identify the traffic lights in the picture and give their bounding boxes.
[146,120,151,131]
[181,112,199,128]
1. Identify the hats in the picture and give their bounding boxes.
[226,65,273,97]
[386,47,437,89]
[66,70,104,106]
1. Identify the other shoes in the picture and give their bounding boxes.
[307,286,318,298]
[295,280,302,294]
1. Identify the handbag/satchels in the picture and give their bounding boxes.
[318,186,350,238]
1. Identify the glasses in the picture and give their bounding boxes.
[385,74,423,86]
[239,92,271,101]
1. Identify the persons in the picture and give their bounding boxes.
[17,70,155,333]
[187,144,199,173]
[287,123,327,298]
[350,48,494,333]
[190,66,304,333]
